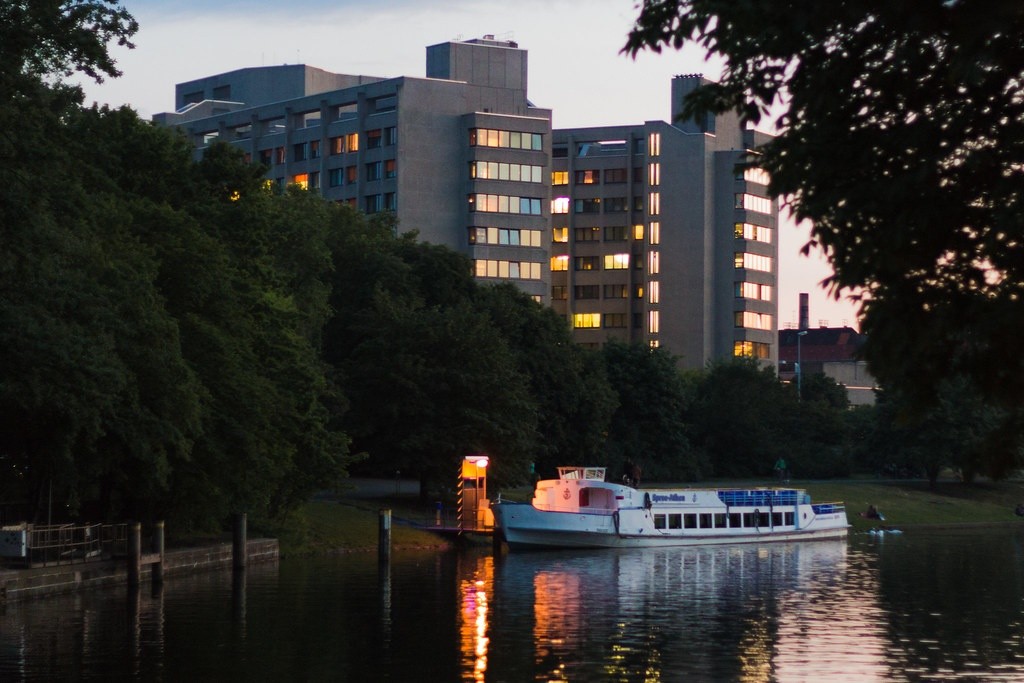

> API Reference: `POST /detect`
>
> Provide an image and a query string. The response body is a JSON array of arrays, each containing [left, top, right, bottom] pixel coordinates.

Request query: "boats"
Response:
[[489, 466, 853, 550]]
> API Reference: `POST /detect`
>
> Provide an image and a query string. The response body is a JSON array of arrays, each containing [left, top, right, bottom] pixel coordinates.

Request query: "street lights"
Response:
[[797, 331, 808, 404]]
